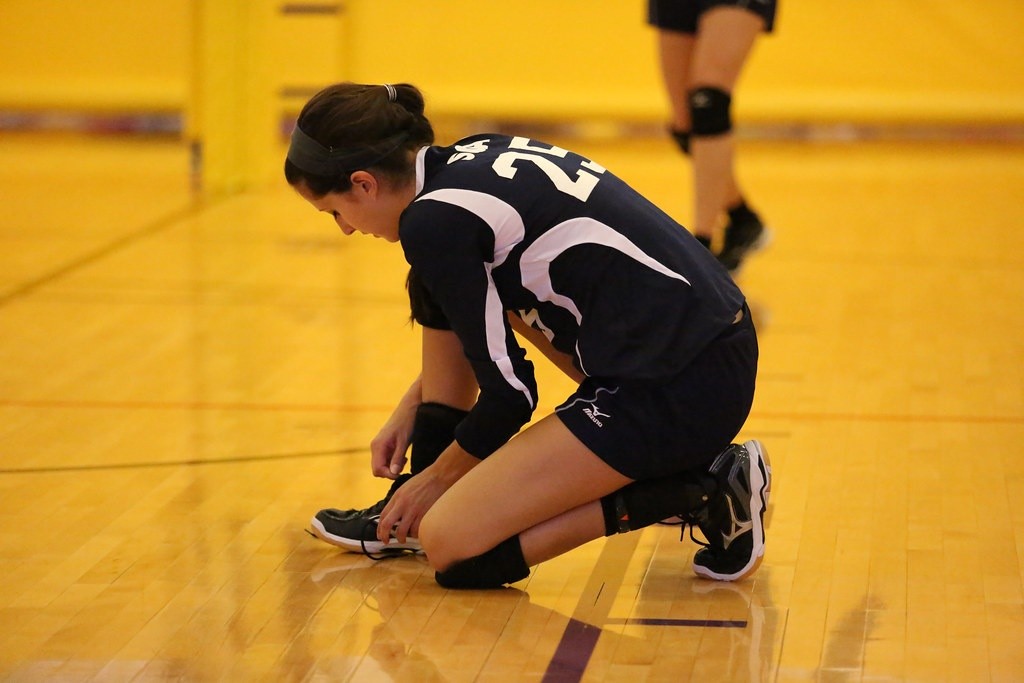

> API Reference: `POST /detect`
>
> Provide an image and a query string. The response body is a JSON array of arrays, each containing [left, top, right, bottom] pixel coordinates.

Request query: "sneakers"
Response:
[[685, 439, 771, 582], [310, 473, 435, 553]]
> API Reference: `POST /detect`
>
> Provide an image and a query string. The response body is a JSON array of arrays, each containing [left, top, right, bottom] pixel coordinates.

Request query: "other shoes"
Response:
[[692, 200, 770, 277]]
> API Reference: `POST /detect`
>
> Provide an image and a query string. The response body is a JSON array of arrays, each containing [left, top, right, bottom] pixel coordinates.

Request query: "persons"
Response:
[[647, 0, 778, 273], [285, 83, 772, 591]]
